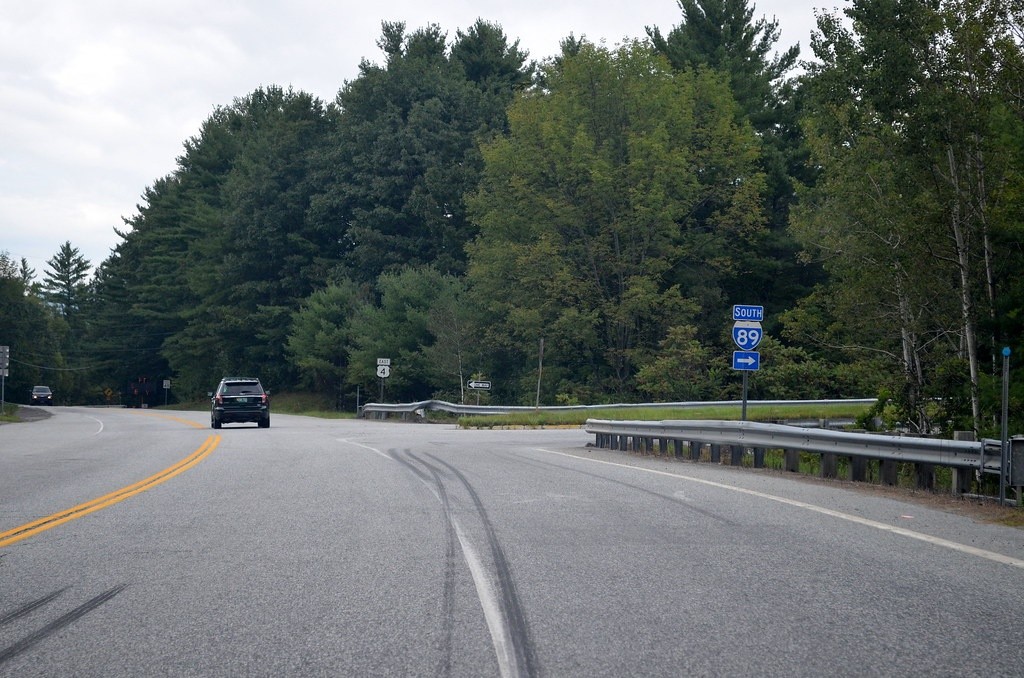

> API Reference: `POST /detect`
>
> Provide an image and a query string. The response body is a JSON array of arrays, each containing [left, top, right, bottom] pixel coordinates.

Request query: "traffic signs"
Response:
[[467, 380, 491, 390]]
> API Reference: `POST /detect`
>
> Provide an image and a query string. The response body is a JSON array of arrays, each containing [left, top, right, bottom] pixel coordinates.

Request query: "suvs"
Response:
[[208, 378, 270, 429], [29, 386, 54, 405]]
